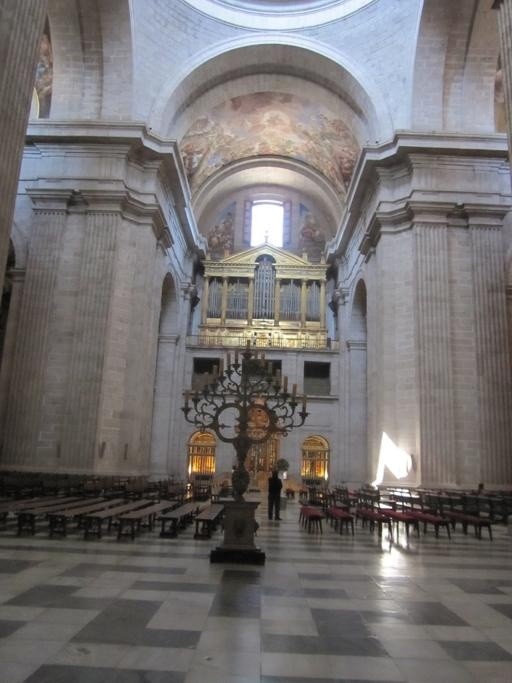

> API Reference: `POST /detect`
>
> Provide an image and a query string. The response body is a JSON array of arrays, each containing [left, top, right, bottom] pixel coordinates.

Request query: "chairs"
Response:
[[286, 481, 511, 542], [1, 469, 265, 543]]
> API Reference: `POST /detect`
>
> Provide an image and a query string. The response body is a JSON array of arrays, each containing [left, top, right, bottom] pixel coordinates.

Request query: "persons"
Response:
[[478, 482, 488, 497], [267, 468, 284, 520]]
